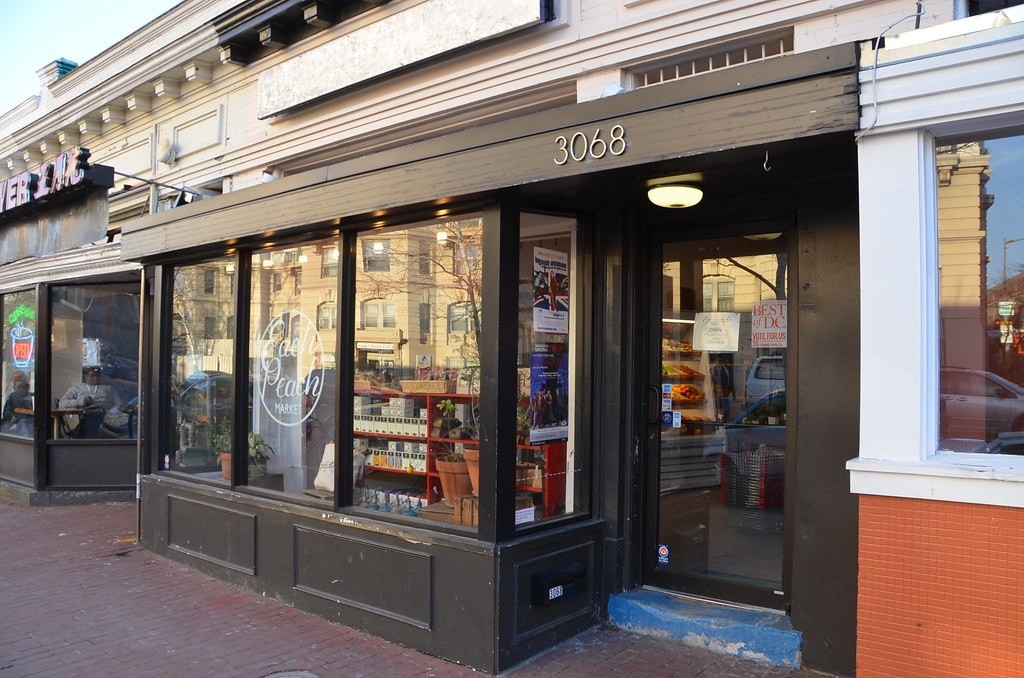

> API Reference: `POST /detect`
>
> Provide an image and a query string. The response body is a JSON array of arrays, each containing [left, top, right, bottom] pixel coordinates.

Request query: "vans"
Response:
[[744, 357, 785, 410]]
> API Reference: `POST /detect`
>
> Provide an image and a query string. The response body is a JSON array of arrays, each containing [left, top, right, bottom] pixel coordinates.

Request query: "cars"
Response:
[[940, 366, 1024, 440], [82, 353, 139, 382], [975, 430, 1024, 455], [703, 388, 786, 488], [304, 369, 336, 409], [179, 375, 313, 441], [99, 392, 142, 439]]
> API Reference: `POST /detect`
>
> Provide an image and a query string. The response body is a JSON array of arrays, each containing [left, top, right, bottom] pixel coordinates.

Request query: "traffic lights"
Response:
[[995, 319, 1013, 325]]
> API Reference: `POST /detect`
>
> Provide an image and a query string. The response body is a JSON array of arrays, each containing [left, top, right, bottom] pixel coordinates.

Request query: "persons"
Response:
[[709, 355, 737, 426], [0, 373, 35, 434], [534, 269, 569, 311], [531, 382, 567, 430], [57, 366, 124, 440]]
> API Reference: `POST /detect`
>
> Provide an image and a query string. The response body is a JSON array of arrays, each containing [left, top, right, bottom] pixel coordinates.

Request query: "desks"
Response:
[[15, 407, 84, 440]]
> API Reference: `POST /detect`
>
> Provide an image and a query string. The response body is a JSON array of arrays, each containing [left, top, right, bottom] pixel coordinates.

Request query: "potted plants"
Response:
[[460, 365, 479, 497], [429, 399, 472, 508]]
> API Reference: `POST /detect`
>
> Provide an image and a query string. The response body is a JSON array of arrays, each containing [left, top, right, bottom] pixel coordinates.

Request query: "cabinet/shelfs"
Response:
[[661, 310, 724, 494], [353, 390, 567, 519]]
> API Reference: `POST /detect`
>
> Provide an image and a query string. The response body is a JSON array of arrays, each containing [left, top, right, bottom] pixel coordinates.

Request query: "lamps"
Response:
[[648, 183, 703, 208]]
